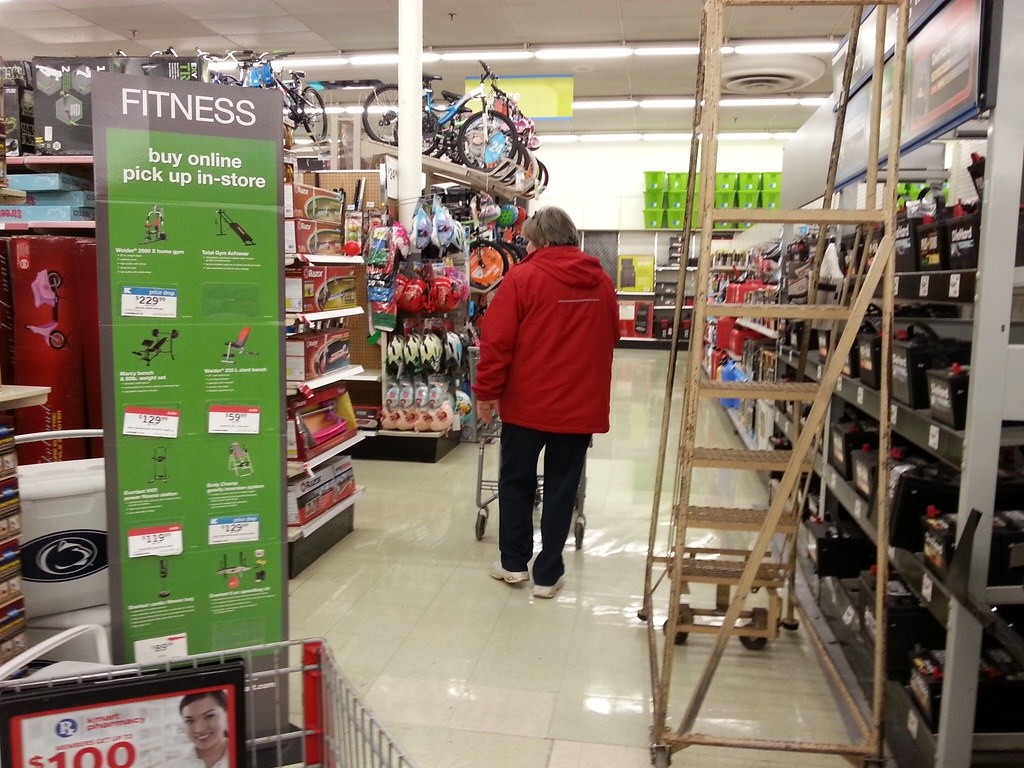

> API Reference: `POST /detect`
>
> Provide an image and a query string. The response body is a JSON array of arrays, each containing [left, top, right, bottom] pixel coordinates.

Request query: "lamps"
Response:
[[30, 33, 844, 108]]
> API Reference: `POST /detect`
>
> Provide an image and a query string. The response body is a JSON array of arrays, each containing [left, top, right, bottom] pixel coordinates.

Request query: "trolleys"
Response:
[[0, 637, 416, 768], [467, 345, 593, 551]]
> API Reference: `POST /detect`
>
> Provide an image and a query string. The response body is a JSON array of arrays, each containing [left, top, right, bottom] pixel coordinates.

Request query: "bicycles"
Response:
[[111, 45, 329, 144], [360, 59, 550, 201]]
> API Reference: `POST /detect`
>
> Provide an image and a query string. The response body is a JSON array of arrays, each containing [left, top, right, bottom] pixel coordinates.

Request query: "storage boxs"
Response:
[[3, 190, 95, 208], [0, 205, 95, 221], [616, 236, 698, 338], [283, 180, 356, 529], [643, 169, 782, 230], [6, 173, 93, 192]]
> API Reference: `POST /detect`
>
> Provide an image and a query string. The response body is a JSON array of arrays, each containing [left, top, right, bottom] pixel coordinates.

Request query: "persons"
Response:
[[179, 690, 228, 768], [472, 205, 621, 598]]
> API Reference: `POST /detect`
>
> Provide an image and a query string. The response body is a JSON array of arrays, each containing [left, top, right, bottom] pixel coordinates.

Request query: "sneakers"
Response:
[[490, 560, 531, 584], [533, 575, 565, 598]]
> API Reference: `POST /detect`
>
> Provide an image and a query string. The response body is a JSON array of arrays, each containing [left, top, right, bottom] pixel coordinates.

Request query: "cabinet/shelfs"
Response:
[[284, 252, 364, 580], [701, 265, 757, 387], [726, 315, 778, 451], [769, 268, 1024, 768], [618, 228, 747, 352], [0, 156, 96, 230]]
